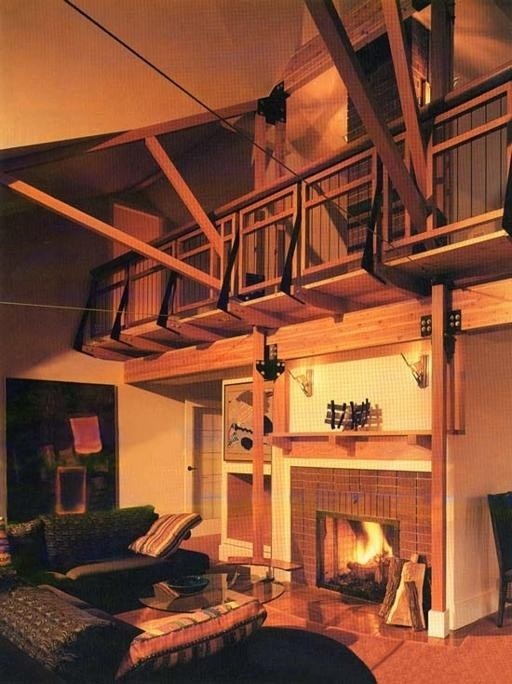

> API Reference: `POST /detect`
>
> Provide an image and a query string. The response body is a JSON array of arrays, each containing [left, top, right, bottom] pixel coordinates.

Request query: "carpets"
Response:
[[127, 626, 376, 684]]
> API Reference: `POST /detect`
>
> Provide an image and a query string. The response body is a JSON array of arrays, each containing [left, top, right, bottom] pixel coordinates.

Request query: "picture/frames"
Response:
[[223, 380, 272, 463]]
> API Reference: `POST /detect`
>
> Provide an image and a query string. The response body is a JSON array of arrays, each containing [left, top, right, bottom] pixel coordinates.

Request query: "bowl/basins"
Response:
[[166, 576, 210, 595]]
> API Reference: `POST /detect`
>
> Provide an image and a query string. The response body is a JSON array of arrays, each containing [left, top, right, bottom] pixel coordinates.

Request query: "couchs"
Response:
[[1, 505, 267, 684]]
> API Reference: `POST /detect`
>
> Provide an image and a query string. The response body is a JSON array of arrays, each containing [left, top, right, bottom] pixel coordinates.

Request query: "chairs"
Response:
[[486, 490, 512, 628]]
